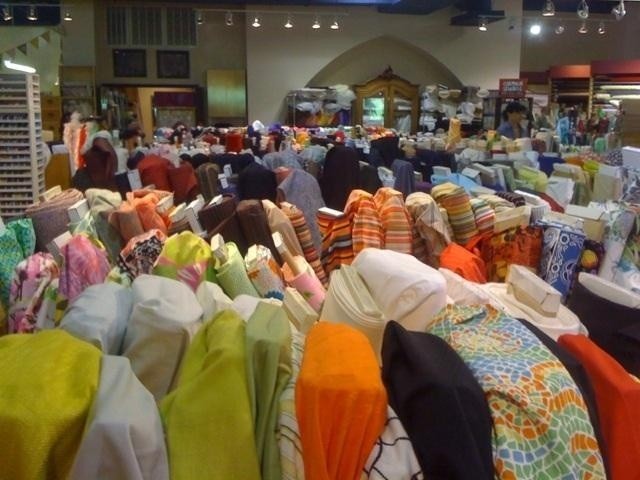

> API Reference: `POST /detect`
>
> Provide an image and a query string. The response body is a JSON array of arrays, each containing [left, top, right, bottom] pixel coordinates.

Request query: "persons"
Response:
[[497, 102, 529, 140]]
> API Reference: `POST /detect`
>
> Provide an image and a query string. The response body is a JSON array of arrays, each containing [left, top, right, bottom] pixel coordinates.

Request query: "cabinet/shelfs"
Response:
[[58, 64, 98, 125], [39, 95, 62, 143], [200, 69, 247, 119]]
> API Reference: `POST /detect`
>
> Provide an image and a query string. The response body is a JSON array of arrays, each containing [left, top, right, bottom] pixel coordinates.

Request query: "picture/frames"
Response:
[[111, 47, 147, 78], [155, 49, 190, 78]]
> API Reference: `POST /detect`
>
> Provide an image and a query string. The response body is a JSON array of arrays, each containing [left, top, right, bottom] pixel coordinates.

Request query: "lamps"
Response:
[[193, 7, 344, 31], [540, 0, 628, 21], [0, 2, 73, 24], [477, 13, 608, 35]]
